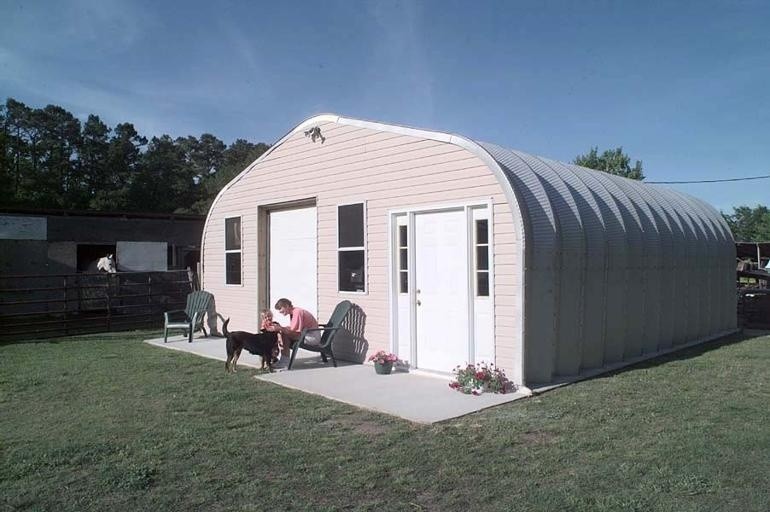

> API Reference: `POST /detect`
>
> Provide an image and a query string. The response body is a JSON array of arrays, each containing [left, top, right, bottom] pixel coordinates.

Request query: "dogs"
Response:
[[221, 318, 280, 375]]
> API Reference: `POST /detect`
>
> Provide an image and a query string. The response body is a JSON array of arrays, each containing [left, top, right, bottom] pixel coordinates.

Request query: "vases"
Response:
[[468, 379, 488, 396], [374, 362, 392, 375]]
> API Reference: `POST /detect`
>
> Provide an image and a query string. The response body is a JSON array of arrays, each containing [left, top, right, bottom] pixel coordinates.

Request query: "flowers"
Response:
[[368, 350, 397, 364], [449, 363, 508, 397]]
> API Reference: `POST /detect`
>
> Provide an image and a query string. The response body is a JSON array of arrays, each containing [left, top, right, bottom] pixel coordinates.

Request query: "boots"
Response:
[[267, 350, 291, 371]]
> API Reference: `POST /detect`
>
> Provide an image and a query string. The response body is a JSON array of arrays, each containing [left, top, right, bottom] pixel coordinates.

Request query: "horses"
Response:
[[78, 253, 117, 274]]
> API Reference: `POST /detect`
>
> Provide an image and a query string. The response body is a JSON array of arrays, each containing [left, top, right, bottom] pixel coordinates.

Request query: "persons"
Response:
[[260, 309, 290, 363], [97, 250, 117, 273], [264, 298, 321, 370]]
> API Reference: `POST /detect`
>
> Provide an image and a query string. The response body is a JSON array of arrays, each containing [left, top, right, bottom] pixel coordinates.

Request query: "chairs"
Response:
[[288, 300, 352, 369], [163, 290, 214, 343]]
